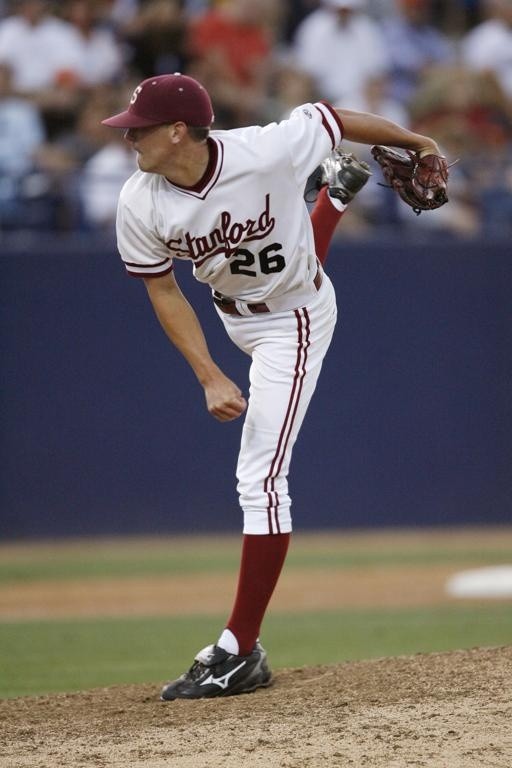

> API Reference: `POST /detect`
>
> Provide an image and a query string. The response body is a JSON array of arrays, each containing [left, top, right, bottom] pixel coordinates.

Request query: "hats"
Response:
[[101, 72, 213, 129]]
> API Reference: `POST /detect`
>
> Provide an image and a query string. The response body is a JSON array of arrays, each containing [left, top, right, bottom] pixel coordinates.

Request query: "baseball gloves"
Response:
[[370, 145, 449, 211]]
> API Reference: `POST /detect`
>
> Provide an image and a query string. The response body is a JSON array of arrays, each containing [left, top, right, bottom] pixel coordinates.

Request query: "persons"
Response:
[[1, 1, 512, 234], [103, 68, 454, 702]]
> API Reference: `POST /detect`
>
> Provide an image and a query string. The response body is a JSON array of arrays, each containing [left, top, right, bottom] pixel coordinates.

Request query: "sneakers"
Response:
[[304, 144, 373, 205], [160, 628, 275, 702]]
